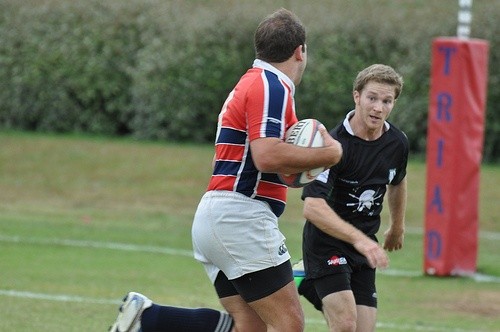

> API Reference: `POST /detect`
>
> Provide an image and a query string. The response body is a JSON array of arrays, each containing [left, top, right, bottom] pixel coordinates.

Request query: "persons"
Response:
[[107, 10, 342, 332], [300, 64, 409, 332]]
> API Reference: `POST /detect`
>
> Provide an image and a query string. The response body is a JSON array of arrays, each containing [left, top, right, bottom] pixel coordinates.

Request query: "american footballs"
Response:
[[277, 118, 328, 189]]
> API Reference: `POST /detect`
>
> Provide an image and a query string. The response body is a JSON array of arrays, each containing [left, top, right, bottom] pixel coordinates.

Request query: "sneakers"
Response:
[[108, 292, 152, 332]]
